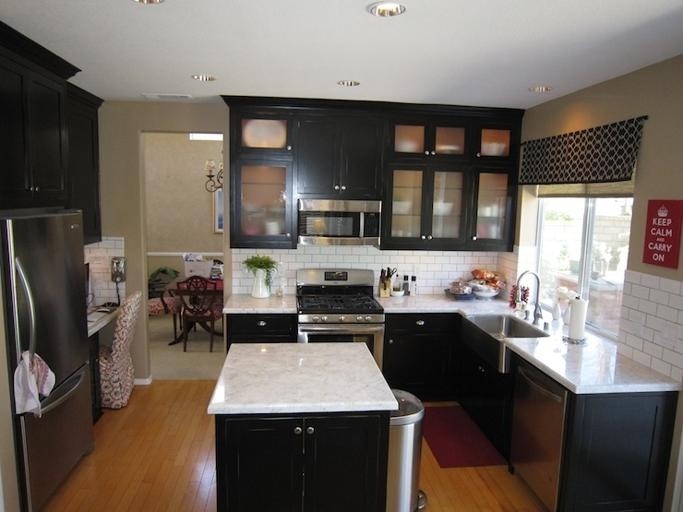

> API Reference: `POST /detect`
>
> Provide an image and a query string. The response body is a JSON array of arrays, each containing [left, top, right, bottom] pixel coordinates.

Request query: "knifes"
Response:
[[380, 267, 392, 289]]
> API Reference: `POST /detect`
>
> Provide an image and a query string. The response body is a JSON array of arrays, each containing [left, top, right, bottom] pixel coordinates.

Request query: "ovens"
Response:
[[298, 321, 386, 373]]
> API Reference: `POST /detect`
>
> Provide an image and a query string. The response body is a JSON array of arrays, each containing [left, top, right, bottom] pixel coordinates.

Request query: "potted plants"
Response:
[[242, 255, 279, 297]]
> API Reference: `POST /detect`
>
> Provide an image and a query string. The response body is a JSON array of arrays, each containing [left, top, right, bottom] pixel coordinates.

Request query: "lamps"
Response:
[[202, 141, 224, 193], [102, 256, 128, 308]]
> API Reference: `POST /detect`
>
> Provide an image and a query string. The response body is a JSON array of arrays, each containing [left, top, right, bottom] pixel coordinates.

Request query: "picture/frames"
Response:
[[212, 183, 223, 235]]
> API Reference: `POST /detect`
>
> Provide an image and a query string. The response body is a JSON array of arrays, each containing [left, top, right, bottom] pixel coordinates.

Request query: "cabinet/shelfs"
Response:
[[382, 312, 458, 402], [458, 314, 515, 464], [67, 80, 105, 246], [471, 105, 525, 252], [381, 100, 472, 252], [220, 93, 298, 249], [0, 20, 82, 211], [298, 98, 381, 201], [223, 313, 298, 353], [207, 410, 399, 512]]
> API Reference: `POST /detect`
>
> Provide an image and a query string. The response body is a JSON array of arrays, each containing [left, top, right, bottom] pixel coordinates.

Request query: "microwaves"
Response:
[[297, 200, 382, 247]]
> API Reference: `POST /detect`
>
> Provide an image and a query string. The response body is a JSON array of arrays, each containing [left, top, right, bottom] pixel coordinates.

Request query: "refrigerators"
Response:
[[1, 206, 97, 512]]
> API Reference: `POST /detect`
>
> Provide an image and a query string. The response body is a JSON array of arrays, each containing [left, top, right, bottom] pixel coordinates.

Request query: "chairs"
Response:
[[175, 277, 216, 352], [98, 290, 144, 409], [147, 282, 182, 339]]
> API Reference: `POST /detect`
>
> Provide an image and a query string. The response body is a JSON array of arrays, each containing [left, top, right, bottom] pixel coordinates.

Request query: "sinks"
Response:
[[465, 313, 551, 342]]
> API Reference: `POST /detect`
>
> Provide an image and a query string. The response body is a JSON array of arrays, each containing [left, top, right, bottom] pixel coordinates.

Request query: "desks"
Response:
[[87, 300, 124, 426], [165, 275, 223, 338]]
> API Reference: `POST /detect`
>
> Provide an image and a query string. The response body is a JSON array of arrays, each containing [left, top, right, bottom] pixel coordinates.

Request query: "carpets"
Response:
[[420, 407, 508, 469]]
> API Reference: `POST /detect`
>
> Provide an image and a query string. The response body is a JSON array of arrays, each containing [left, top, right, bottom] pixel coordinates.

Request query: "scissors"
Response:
[[389, 268, 397, 277]]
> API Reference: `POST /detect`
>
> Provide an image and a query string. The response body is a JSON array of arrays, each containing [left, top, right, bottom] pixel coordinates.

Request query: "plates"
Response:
[[435, 144, 461, 153]]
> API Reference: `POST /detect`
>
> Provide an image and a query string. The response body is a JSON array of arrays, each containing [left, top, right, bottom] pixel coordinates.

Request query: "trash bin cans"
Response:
[[385, 388, 427, 512]]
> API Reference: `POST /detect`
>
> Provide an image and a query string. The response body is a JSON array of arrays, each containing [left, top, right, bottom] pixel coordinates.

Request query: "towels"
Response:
[[13, 350, 57, 419]]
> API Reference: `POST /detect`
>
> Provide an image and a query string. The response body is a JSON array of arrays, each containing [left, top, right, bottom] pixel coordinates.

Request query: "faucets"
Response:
[[513, 270, 543, 325]]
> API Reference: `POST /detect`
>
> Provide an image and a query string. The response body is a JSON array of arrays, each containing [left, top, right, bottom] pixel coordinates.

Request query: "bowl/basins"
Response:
[[392, 200, 411, 215], [432, 202, 454, 216], [482, 141, 505, 155]]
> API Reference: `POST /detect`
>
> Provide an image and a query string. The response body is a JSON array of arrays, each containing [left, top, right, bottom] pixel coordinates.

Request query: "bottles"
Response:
[[393, 274, 417, 297]]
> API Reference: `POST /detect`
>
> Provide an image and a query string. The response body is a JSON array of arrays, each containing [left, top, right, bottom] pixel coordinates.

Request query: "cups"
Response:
[[481, 204, 499, 217]]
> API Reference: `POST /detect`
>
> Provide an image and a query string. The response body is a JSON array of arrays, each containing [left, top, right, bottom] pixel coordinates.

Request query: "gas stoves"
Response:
[[297, 289, 384, 324]]
